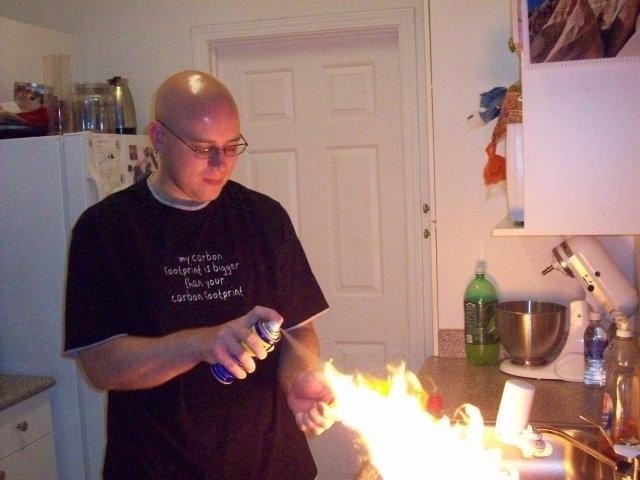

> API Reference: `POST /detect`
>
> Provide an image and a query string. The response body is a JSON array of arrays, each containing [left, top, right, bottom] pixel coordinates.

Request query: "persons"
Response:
[[59, 69, 339, 479]]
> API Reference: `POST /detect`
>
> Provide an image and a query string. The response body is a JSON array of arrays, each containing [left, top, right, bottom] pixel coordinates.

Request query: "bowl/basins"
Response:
[[13, 80, 50, 113]]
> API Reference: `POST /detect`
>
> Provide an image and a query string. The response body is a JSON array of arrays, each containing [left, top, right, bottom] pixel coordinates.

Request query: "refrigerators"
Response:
[[1, 132, 159, 480]]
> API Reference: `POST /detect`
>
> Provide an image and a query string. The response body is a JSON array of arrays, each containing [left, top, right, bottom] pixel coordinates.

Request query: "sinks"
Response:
[[357, 421, 618, 480]]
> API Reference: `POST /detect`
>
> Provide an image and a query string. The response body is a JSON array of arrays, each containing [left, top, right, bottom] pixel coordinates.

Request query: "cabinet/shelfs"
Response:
[[492, 1, 640, 242], [0, 390, 57, 479]]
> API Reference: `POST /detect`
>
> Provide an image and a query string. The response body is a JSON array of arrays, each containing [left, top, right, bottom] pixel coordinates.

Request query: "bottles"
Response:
[[463, 268, 498, 363], [494, 237, 638, 382], [583, 312, 608, 387], [71, 82, 101, 132]]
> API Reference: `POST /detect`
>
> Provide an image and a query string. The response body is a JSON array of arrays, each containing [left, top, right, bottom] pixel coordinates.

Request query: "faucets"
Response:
[[532, 426, 634, 474]]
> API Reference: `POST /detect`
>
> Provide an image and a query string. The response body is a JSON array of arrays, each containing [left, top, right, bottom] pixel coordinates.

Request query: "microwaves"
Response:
[[505, 122, 524, 227]]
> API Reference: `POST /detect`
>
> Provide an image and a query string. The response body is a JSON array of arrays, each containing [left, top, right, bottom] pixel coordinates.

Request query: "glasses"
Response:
[[154, 116, 248, 160]]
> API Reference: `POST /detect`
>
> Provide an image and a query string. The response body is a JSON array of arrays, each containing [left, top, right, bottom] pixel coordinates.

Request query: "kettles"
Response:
[[101, 76, 137, 135]]
